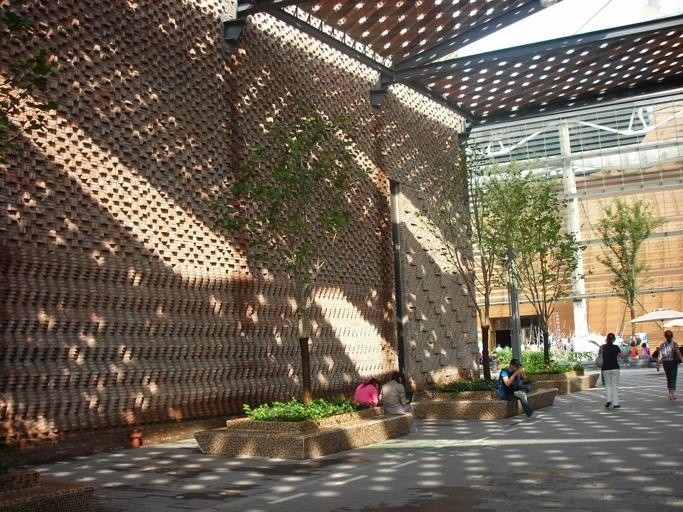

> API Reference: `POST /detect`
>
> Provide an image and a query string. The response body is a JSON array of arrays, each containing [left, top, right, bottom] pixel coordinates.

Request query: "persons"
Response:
[[496, 359, 533, 417], [487, 338, 574, 355], [621, 334, 682, 363], [597, 332, 623, 409], [379, 372, 418, 432], [352, 377, 378, 407], [656, 330, 682, 400]]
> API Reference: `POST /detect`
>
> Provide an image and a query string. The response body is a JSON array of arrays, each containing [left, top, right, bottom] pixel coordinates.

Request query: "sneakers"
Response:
[[605, 402, 611, 407], [669, 394, 677, 400], [528, 414, 541, 420], [513, 391, 526, 399], [614, 406, 620, 407]]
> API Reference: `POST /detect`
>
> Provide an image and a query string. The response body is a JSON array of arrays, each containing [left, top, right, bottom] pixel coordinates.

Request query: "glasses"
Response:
[[513, 364, 519, 368]]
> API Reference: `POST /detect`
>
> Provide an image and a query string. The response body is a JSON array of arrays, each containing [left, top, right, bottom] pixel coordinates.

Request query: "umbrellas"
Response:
[[627, 306, 683, 323], [662, 319, 683, 327]]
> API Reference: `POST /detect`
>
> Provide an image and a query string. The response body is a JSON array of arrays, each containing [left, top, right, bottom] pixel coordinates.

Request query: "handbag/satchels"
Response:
[[648, 351, 649, 355], [673, 351, 681, 363], [503, 384, 515, 394], [596, 354, 603, 368]]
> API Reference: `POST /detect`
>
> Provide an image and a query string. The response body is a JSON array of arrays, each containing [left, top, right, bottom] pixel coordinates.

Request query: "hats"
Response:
[[511, 359, 520, 365]]
